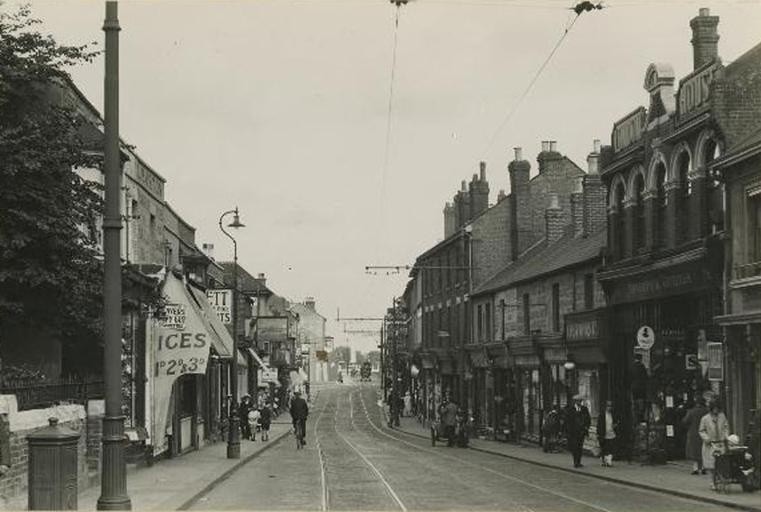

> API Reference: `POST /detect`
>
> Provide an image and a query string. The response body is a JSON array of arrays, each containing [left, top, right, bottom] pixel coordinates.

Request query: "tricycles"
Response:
[[431, 411, 469, 447]]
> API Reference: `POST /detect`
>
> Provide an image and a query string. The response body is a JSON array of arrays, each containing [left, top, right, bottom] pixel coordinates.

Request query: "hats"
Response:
[[726, 435, 739, 444], [572, 394, 582, 400]]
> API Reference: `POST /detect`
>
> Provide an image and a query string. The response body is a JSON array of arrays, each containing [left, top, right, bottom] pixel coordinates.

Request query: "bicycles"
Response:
[[294, 417, 306, 449]]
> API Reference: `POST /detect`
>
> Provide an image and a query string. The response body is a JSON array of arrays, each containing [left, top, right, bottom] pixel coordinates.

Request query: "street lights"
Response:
[[221, 208, 245, 458]]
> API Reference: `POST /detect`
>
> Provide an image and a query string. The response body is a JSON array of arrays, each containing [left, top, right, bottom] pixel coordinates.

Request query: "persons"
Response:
[[564, 395, 591, 468], [594, 401, 623, 466], [385, 391, 402, 427], [724, 433, 752, 464], [440, 395, 457, 447], [289, 391, 309, 444], [679, 402, 707, 476], [698, 400, 731, 469], [437, 397, 449, 416], [238, 386, 272, 442], [541, 404, 561, 452]]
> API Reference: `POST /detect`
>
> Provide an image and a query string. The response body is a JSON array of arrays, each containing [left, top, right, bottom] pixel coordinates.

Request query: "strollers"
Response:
[[709, 435, 760, 493]]
